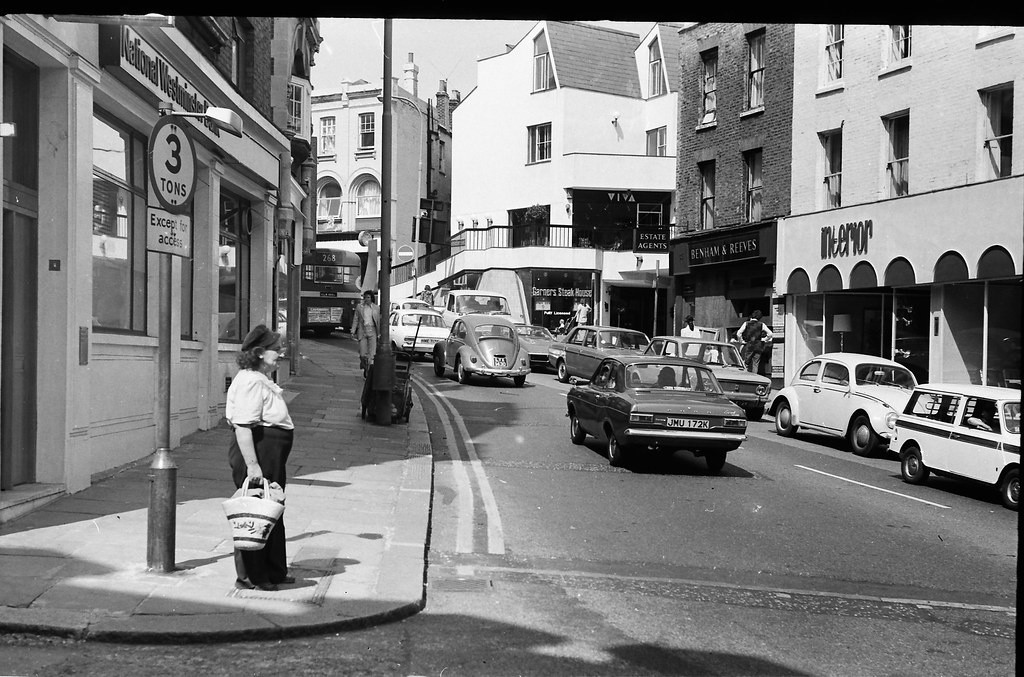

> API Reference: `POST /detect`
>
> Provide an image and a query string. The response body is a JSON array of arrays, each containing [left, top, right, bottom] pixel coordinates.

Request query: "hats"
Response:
[[241, 324, 281, 350]]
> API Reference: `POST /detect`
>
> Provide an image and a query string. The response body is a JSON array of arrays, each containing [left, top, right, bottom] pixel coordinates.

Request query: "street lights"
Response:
[[378, 93, 420, 300], [145, 100, 246, 568]]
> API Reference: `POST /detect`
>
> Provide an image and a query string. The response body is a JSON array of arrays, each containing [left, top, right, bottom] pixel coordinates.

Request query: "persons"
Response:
[[736, 310, 773, 374], [318, 268, 332, 281], [465, 295, 480, 305], [350, 289, 381, 381], [572, 297, 592, 340], [488, 301, 503, 312], [420, 285, 434, 306], [225, 324, 296, 591], [680, 315, 702, 356], [654, 367, 677, 388], [966, 403, 1000, 434], [551, 319, 565, 332]]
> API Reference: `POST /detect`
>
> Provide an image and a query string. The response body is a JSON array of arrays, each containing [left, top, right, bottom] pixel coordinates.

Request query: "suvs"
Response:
[[888, 386, 1020, 512]]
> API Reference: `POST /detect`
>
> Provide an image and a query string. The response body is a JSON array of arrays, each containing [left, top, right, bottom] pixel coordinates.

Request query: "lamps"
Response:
[[566, 204, 570, 211], [612, 114, 621, 127], [635, 255, 643, 266]]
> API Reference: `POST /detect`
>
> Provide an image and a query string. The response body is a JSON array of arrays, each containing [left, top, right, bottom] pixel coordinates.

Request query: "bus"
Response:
[[300, 247, 363, 338]]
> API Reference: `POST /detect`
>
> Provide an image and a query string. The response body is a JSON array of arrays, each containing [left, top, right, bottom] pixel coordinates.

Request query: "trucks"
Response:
[[447, 292, 511, 321]]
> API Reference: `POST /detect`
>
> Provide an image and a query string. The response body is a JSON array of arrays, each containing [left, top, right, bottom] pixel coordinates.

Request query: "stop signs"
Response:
[[398, 245, 415, 262]]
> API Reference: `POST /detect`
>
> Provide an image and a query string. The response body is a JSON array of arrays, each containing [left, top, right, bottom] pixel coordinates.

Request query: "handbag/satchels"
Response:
[[226, 476, 285, 550]]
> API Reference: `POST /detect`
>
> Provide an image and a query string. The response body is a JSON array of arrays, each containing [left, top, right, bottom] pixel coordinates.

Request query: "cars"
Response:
[[549, 321, 656, 383], [569, 356, 747, 471], [645, 337, 771, 423], [391, 299, 429, 314], [432, 314, 534, 386], [391, 308, 450, 356], [769, 353, 918, 457], [513, 324, 555, 372]]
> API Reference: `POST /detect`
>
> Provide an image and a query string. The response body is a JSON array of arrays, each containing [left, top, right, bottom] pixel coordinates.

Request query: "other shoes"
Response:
[[236, 575, 295, 591]]
